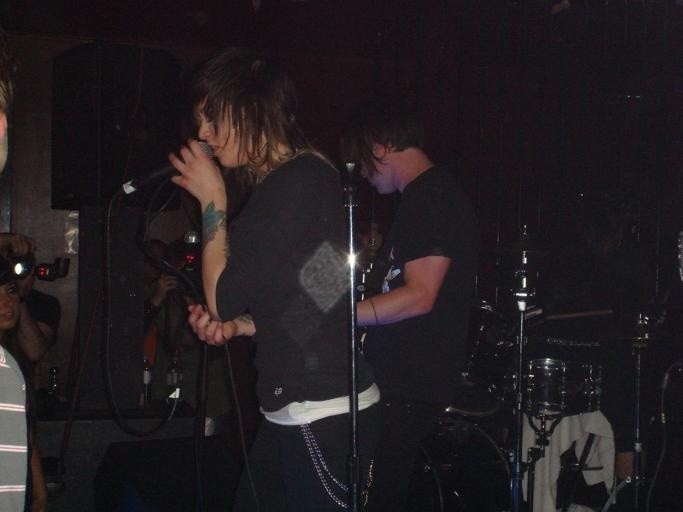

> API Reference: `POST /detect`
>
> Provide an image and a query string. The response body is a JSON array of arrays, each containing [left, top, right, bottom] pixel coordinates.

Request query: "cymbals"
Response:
[[607, 337, 674, 351]]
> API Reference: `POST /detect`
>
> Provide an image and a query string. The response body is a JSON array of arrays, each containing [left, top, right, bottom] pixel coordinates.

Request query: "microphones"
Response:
[[122, 141, 213, 195]]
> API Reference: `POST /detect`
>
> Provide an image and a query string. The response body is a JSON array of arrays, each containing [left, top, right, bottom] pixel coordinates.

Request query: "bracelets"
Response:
[[366, 295, 380, 330]]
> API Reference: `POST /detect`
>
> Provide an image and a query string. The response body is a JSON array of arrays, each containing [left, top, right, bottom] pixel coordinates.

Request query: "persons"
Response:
[[0, 233, 64, 386], [350, 211, 397, 299], [0, 32, 34, 512], [339, 94, 484, 508], [0, 255, 50, 511], [177, 39, 388, 510], [127, 258, 238, 418]]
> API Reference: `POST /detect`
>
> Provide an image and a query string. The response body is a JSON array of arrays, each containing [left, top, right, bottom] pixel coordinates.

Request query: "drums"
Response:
[[516, 360, 600, 417], [447, 304, 525, 417], [404, 422, 515, 511]]
